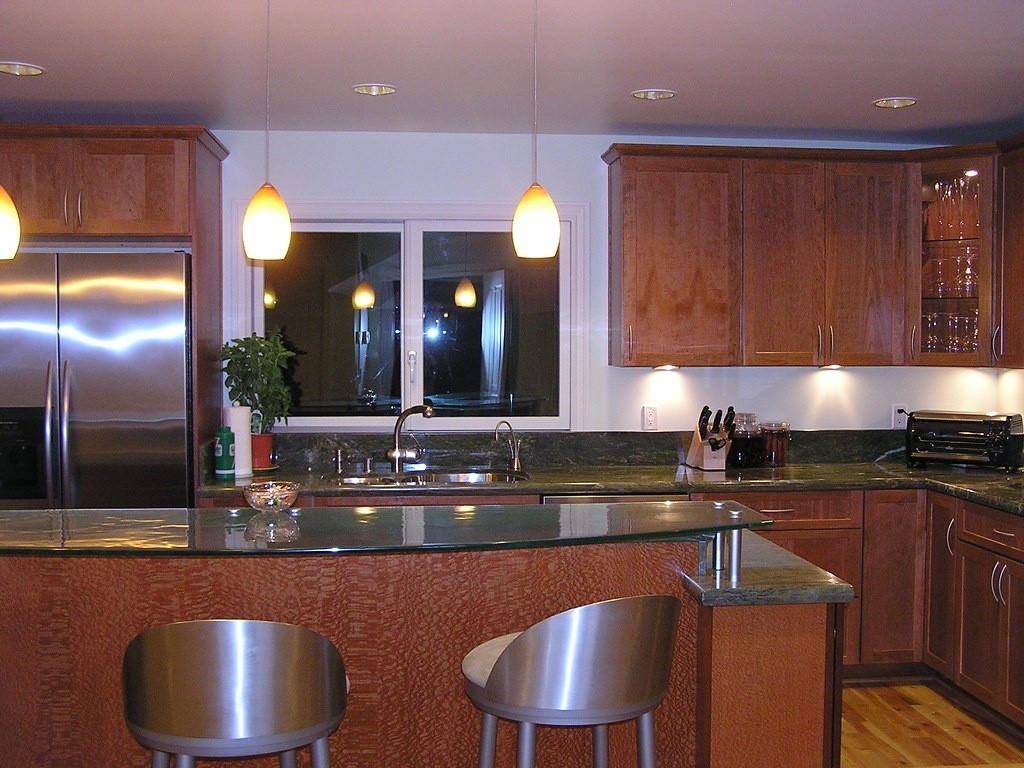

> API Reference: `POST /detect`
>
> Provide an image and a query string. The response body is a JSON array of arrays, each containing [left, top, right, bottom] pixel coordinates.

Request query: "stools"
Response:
[[123, 619, 352, 768], [461, 593, 681, 768]]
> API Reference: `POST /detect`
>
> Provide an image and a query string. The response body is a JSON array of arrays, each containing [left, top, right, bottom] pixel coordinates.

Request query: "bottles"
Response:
[[215, 427, 235, 480], [762, 422, 790, 466], [731, 413, 761, 465]]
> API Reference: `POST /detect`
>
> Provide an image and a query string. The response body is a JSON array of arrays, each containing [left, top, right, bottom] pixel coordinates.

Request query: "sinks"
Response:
[[334, 477, 398, 488], [401, 471, 529, 487]]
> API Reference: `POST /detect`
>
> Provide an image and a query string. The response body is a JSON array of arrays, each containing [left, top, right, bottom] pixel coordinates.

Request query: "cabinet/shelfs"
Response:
[[702, 491, 1024, 734], [601, 141, 1024, 369], [0, 121, 228, 244]]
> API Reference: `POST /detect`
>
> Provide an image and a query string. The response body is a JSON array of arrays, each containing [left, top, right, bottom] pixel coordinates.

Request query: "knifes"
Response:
[[697, 406, 736, 438]]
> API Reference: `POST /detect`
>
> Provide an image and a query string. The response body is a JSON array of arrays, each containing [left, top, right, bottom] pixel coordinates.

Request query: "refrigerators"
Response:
[[0, 248, 191, 510]]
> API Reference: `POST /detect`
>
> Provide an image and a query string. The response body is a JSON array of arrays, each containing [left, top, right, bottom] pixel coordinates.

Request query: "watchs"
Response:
[[449, 373, 451, 375]]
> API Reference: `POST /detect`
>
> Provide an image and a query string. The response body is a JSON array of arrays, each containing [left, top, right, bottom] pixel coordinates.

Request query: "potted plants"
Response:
[[217, 327, 296, 468]]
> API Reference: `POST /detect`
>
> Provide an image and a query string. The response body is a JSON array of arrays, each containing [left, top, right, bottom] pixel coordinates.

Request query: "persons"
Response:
[[424, 281, 454, 398]]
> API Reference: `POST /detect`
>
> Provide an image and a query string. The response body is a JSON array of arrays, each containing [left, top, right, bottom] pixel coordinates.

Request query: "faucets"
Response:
[[494, 420, 523, 470], [385, 404, 435, 472]]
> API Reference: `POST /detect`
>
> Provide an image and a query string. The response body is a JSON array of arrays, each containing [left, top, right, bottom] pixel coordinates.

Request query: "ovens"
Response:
[[907, 410, 1024, 472]]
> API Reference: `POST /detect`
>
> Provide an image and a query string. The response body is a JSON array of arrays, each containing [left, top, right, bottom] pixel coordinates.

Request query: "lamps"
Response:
[[242, 0, 291, 261], [455, 234, 476, 308], [511, 0, 561, 261], [264, 283, 277, 308], [353, 268, 375, 308]]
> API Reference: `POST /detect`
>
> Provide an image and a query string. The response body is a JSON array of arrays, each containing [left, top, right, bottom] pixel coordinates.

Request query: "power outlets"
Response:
[[891, 404, 908, 429], [643, 406, 658, 430]]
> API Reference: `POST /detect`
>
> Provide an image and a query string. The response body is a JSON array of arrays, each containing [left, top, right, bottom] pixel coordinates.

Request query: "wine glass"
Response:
[[923, 176, 978, 353]]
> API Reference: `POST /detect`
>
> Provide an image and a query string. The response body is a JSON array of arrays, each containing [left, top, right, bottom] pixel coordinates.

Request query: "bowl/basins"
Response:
[[242, 484, 298, 512], [245, 513, 301, 541]]
